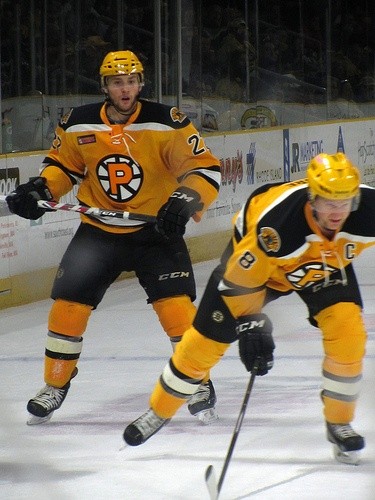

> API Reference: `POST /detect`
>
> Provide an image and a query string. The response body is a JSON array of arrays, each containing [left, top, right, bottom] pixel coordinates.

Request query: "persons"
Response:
[[6, 50, 221, 426], [118, 153, 375, 466]]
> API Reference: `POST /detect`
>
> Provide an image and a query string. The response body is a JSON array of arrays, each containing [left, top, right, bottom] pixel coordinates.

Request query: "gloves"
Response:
[[156, 185, 204, 241], [5, 177, 57, 220], [235, 313, 275, 376]]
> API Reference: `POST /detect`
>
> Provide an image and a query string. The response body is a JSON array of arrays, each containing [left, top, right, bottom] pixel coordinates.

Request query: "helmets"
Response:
[[306, 152, 360, 199], [100, 50, 144, 76]]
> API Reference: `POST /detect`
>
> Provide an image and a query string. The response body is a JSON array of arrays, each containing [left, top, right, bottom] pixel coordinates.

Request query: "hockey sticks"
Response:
[[204, 362, 259, 499], [0, 193, 159, 224]]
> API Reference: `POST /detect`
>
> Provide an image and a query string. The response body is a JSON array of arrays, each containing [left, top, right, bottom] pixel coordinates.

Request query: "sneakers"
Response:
[[119, 407, 171, 452], [188, 379, 217, 425], [326, 421, 364, 465], [26, 366, 78, 425]]
